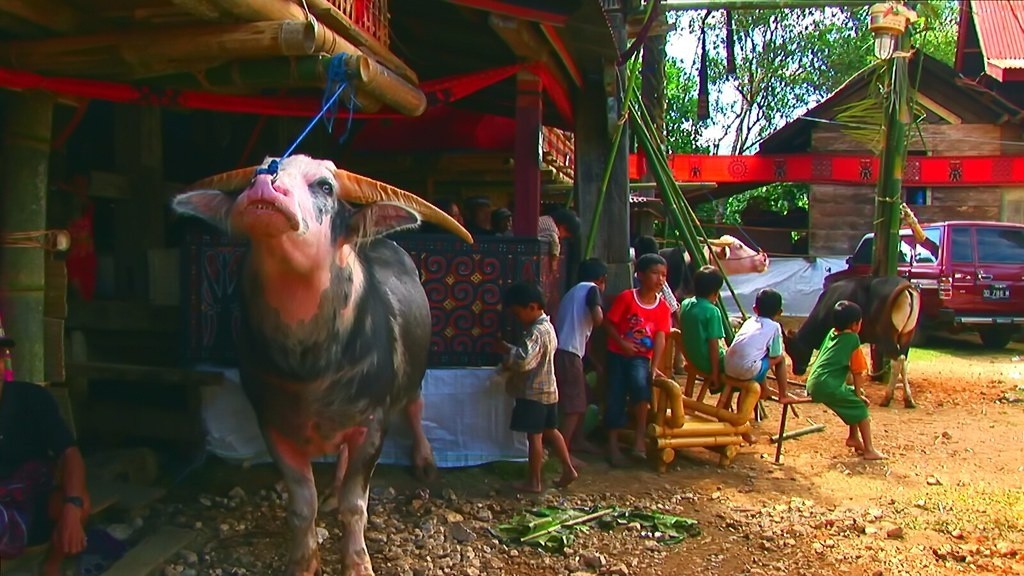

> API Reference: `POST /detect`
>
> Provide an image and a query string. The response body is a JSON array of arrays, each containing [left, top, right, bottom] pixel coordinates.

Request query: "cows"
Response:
[[172, 153, 474, 576], [780, 275, 921, 408], [627, 230, 769, 375]]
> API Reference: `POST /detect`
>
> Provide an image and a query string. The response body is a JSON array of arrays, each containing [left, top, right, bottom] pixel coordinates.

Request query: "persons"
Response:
[[0, 379, 88, 576], [554, 258, 609, 468], [680, 265, 741, 396], [467, 205, 525, 258], [724, 288, 800, 404], [537, 209, 581, 242], [806, 300, 890, 460], [603, 253, 672, 468], [633, 236, 681, 327], [491, 281, 578, 492], [432, 199, 464, 225]]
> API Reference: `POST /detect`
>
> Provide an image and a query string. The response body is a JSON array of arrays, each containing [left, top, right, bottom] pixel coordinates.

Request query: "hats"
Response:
[[492, 209, 512, 226], [0, 324, 15, 346]]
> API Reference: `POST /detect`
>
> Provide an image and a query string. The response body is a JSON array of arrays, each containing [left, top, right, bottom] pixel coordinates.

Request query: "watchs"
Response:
[[63, 496, 84, 508]]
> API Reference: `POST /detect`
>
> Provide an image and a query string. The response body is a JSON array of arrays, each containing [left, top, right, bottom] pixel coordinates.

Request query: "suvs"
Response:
[[822, 220, 1024, 348]]
